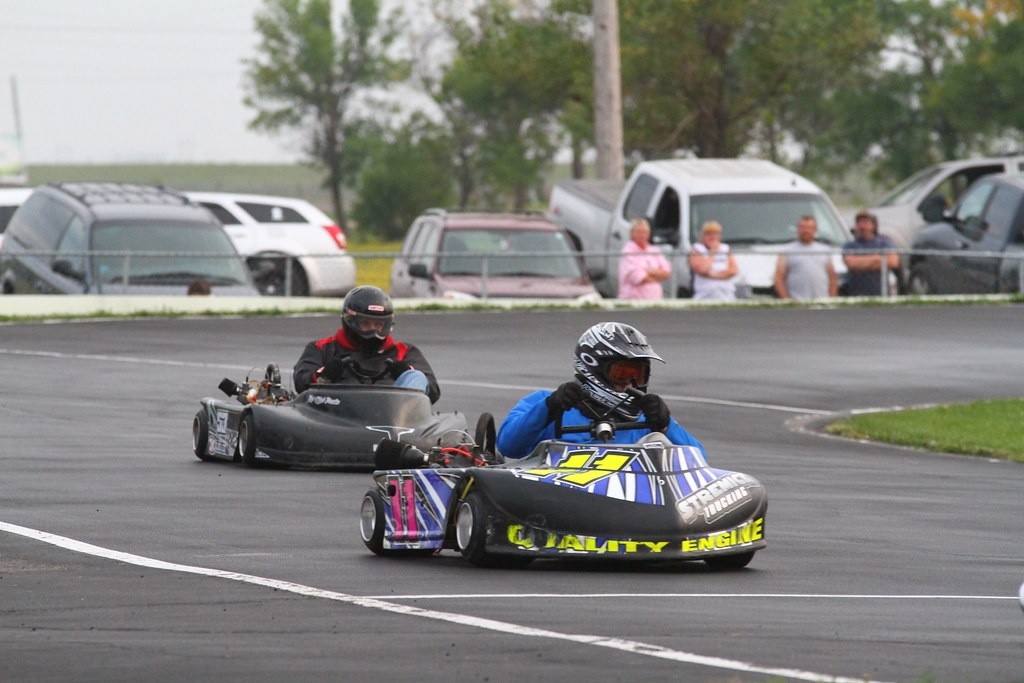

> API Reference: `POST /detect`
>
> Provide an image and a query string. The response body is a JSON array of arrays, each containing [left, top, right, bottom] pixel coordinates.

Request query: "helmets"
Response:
[[573, 322, 667, 422], [341, 285, 396, 355]]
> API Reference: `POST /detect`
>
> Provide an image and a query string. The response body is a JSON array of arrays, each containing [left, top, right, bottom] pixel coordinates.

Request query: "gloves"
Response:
[[387, 360, 412, 382], [636, 394, 672, 432], [319, 354, 347, 382], [547, 382, 585, 414]]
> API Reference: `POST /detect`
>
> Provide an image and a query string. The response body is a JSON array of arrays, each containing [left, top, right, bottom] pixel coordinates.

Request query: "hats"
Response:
[[628, 218, 651, 232], [701, 220, 722, 232]]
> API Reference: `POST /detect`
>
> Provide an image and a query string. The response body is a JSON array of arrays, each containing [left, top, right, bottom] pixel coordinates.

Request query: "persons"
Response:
[[187, 279, 210, 296], [293, 285, 439, 406], [841, 210, 900, 299], [616, 219, 671, 299], [497, 320, 710, 467], [689, 220, 739, 298], [775, 215, 837, 298]]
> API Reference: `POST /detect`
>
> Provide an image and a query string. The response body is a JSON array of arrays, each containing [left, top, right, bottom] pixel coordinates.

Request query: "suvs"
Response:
[[2, 180, 264, 297], [390, 207, 604, 302], [175, 190, 357, 295]]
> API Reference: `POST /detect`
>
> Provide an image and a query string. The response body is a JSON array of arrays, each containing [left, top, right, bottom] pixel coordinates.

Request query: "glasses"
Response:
[[596, 358, 650, 386]]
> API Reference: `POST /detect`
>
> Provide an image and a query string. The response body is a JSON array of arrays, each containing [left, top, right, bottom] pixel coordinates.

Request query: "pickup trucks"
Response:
[[545, 147, 858, 299]]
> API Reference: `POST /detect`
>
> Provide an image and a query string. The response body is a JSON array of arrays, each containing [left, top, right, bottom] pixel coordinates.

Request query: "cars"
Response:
[[843, 156, 1024, 284], [905, 171, 1024, 295]]
